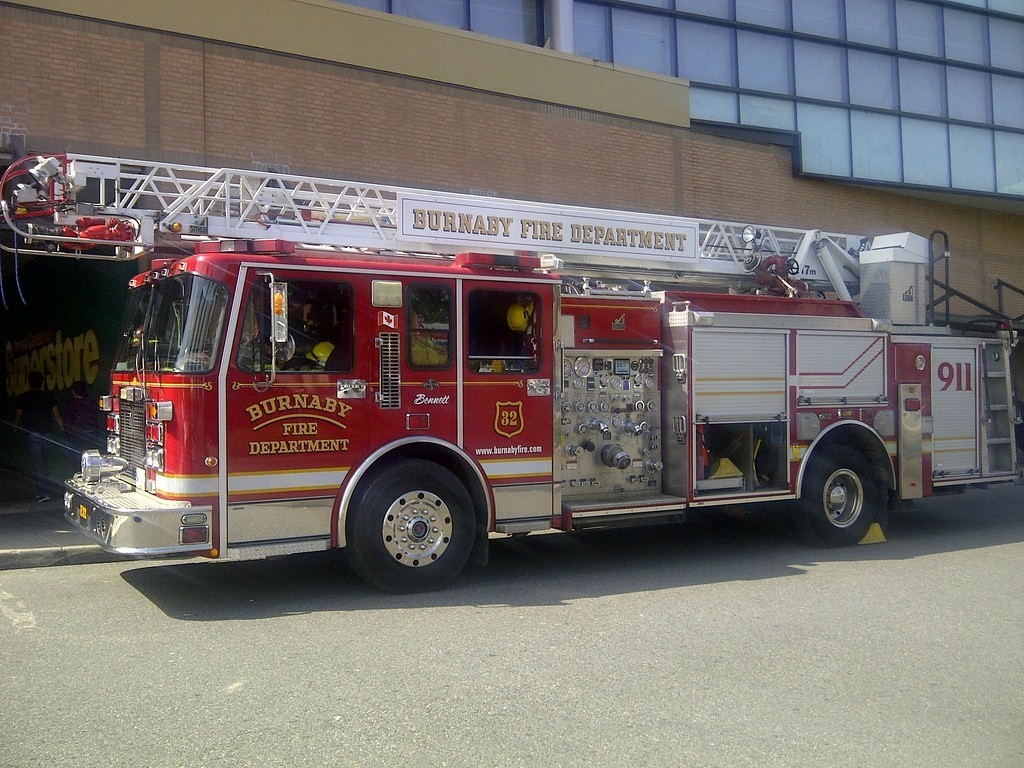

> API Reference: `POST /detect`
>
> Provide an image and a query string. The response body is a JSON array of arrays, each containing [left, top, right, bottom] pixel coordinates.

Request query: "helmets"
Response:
[[507, 301, 533, 331], [306, 342, 333, 367]]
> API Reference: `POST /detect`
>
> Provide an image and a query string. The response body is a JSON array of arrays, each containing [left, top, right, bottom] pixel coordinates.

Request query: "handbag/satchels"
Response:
[[64, 438, 83, 464]]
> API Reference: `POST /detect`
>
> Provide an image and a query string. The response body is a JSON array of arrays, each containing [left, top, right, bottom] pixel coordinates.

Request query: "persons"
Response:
[[61, 381, 100, 476], [12, 370, 65, 504]]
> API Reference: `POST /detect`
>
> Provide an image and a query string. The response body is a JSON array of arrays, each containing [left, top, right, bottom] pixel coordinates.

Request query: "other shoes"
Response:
[[37, 496, 50, 502], [32, 495, 39, 501]]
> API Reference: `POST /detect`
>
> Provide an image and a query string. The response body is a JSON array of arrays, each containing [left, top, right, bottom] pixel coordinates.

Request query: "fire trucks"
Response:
[[1, 153, 1024, 596]]
